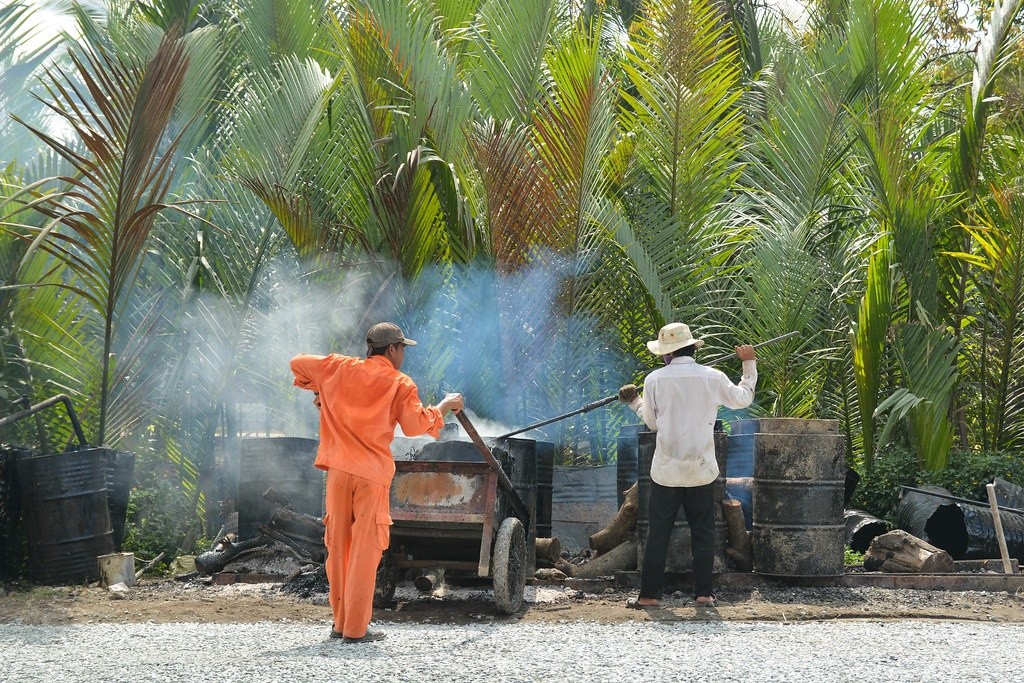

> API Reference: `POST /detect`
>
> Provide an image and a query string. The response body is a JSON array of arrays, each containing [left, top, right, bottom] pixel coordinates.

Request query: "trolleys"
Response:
[[316, 401, 531, 616]]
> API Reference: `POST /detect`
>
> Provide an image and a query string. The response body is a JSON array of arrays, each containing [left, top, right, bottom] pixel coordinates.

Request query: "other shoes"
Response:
[[329, 624, 343, 638], [343, 631, 387, 643]]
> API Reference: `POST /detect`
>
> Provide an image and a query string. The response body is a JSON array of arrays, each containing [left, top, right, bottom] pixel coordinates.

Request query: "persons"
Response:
[[291, 321, 463, 644], [620, 322, 758, 609]]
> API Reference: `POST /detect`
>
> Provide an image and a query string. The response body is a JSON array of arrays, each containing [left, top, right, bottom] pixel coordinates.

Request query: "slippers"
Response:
[[696, 592, 718, 607], [625, 595, 659, 610]]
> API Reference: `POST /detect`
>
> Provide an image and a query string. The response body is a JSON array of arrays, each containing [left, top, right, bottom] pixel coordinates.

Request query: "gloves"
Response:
[[618, 383, 637, 404]]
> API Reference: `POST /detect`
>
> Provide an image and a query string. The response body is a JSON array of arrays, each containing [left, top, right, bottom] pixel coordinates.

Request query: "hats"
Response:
[[366, 322, 417, 358], [647, 322, 706, 356]]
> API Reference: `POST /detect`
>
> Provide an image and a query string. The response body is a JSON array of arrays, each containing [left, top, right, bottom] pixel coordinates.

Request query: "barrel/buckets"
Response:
[[387, 438, 617, 542], [618, 416, 848, 576], [5, 444, 136, 587], [240, 438, 323, 535]]
